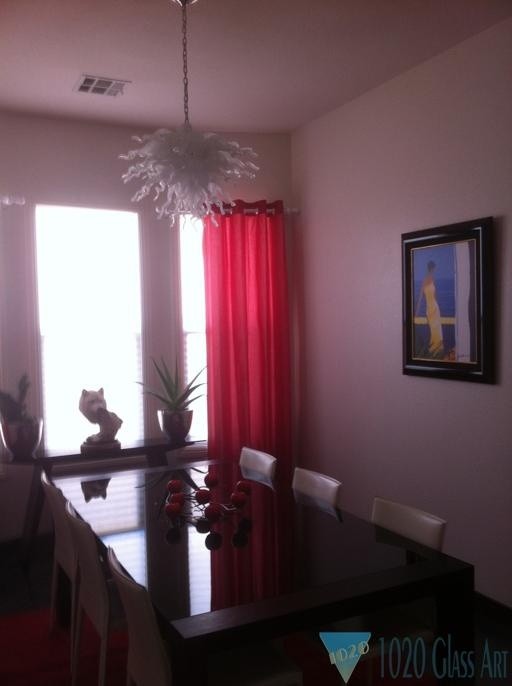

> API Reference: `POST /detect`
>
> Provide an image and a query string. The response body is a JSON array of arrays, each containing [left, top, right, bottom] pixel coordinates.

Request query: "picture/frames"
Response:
[[401, 216, 496, 385]]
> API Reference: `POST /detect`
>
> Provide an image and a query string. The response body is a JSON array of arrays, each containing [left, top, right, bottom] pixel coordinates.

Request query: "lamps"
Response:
[[117, 0, 262, 229]]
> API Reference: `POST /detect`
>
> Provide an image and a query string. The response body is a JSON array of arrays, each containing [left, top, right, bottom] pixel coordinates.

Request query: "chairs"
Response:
[[65, 511, 127, 686], [372, 496, 447, 552], [239, 447, 277, 476], [290, 467, 342, 504], [40, 468, 72, 637], [107, 544, 199, 685]]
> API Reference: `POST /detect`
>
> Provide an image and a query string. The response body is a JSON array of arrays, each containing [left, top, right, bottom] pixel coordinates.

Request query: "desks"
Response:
[[50, 456, 476, 684]]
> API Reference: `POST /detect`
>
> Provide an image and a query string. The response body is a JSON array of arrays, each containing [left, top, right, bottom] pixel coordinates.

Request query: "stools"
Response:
[[284, 614, 436, 684]]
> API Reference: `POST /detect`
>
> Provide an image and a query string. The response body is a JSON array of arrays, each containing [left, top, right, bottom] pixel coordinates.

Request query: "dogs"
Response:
[[79, 388, 106, 422]]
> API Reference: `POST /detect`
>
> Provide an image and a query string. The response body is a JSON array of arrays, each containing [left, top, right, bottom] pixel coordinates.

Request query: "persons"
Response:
[[414, 261, 444, 358]]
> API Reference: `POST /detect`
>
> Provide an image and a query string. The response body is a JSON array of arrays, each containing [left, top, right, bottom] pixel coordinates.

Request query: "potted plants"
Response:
[[1, 374, 45, 462], [133, 354, 208, 442]]
